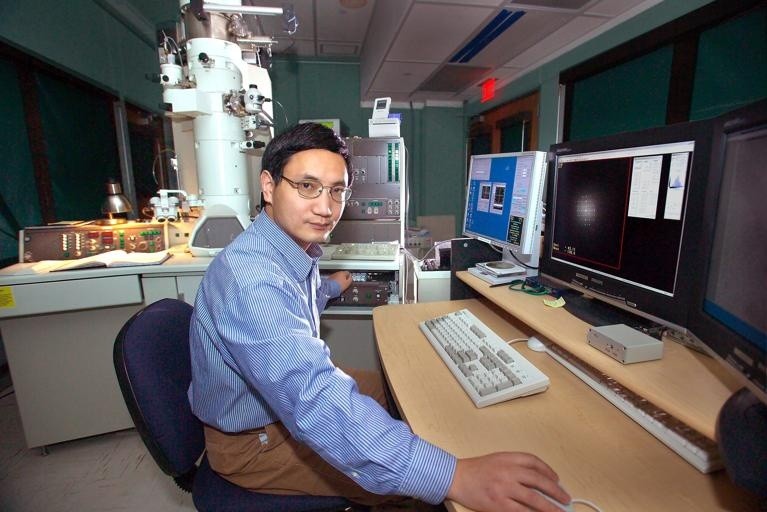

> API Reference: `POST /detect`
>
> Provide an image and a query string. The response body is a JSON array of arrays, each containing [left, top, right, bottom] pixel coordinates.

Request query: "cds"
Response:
[[486, 262, 515, 269]]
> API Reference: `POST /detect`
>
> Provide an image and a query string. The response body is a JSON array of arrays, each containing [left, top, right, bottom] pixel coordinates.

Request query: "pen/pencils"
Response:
[[489, 279, 523, 288]]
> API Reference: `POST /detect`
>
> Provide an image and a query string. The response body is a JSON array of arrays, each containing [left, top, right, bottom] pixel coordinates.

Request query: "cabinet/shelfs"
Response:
[[0, 268, 177, 456], [172, 263, 402, 373]]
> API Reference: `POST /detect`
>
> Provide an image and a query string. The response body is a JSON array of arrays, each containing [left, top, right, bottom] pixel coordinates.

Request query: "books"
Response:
[[50, 250, 174, 272]]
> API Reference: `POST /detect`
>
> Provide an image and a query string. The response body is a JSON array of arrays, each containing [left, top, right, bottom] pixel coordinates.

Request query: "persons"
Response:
[[186, 122, 572, 512]]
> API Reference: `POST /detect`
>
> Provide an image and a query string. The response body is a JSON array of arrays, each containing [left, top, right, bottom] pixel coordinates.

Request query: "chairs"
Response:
[[112, 298, 376, 512]]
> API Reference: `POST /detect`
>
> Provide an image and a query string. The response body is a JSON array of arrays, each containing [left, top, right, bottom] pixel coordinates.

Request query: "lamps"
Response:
[[95, 180, 133, 226]]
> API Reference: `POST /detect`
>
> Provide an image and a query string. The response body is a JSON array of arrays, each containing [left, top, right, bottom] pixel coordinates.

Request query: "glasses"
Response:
[[273, 173, 354, 203]]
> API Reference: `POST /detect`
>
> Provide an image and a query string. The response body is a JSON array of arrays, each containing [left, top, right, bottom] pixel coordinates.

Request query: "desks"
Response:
[[372, 269, 765, 511]]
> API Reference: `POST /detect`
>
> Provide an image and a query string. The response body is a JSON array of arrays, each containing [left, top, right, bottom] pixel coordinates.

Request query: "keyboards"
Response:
[[547, 344, 728, 474], [419, 308, 549, 408]]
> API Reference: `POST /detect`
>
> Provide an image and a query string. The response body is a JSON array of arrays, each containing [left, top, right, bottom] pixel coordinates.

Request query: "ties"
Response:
[[309, 263, 323, 341]]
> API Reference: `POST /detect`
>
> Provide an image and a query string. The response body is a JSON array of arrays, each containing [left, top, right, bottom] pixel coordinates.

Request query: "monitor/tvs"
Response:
[[539, 120, 712, 329], [463, 151, 547, 287], [690, 98, 766, 393]]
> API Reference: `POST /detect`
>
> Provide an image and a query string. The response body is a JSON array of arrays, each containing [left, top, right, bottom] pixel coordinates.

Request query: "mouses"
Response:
[[528, 482, 575, 512], [528, 332, 552, 354]]
